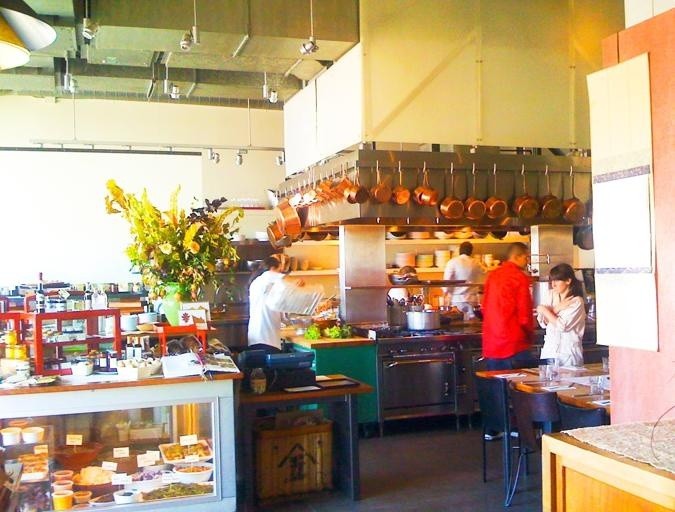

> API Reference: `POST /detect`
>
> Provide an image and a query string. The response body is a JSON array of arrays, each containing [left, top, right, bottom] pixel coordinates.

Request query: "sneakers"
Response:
[[510, 427, 519, 438], [484, 429, 506, 441]]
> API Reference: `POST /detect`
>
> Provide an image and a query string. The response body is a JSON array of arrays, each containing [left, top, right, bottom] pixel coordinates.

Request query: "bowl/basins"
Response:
[[120, 313, 157, 332], [70, 363, 94, 377], [1, 420, 215, 505], [387, 305, 425, 327], [291, 317, 313, 335], [315, 319, 337, 329], [232, 234, 245, 241], [116, 366, 150, 380], [256, 231, 270, 242]]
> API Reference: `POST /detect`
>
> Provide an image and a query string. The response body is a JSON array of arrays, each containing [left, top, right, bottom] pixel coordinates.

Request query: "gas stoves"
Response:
[[366, 326, 467, 355]]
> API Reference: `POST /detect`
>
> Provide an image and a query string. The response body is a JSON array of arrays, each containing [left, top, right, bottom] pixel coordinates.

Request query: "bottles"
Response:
[[147, 300, 154, 312], [250, 368, 266, 394], [585, 295, 597, 324], [36, 273, 107, 313], [46, 335, 157, 369]]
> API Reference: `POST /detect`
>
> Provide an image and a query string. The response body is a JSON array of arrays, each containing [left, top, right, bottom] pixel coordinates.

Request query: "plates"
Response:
[[309, 266, 323, 270], [396, 244, 501, 268]]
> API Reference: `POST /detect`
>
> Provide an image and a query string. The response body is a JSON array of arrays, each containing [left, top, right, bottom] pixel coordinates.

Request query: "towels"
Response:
[[560, 418, 675, 479]]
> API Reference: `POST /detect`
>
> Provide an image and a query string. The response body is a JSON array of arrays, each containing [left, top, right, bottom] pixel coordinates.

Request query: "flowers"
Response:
[[102, 178, 249, 302]]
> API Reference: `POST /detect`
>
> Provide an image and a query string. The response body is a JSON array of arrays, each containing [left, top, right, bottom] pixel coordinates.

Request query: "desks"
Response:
[[541, 420, 675, 512], [279, 324, 380, 438], [237, 374, 374, 511]]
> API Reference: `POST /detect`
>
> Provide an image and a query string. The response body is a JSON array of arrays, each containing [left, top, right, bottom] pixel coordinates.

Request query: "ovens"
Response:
[[381, 355, 456, 407]]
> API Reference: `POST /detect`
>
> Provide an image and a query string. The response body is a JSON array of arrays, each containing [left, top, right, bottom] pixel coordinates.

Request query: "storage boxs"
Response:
[[254, 413, 334, 509]]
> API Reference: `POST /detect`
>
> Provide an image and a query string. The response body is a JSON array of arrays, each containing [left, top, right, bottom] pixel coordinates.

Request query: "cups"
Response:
[[601, 356, 609, 373], [51, 470, 92, 511], [547, 353, 561, 381], [300, 259, 309, 270], [290, 256, 298, 271], [590, 375, 603, 395], [539, 365, 547, 381]]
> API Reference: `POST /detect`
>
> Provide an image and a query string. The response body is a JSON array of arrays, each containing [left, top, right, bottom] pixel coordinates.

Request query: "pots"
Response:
[[247, 260, 266, 271], [406, 310, 440, 330], [215, 259, 240, 272], [267, 161, 597, 250]]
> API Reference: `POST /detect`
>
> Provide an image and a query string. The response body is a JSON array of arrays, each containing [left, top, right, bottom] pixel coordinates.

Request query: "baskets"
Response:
[[251, 411, 335, 500]]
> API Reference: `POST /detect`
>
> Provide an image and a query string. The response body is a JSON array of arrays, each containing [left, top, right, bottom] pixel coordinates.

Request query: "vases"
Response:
[[161, 281, 190, 327]]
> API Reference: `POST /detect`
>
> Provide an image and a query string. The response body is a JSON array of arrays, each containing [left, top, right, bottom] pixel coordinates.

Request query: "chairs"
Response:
[[474, 361, 611, 507]]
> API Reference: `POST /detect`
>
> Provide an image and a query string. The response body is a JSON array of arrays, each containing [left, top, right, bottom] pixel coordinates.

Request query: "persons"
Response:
[[246, 254, 293, 351], [481, 243, 539, 440], [532, 263, 586, 366], [441, 241, 483, 313]]
